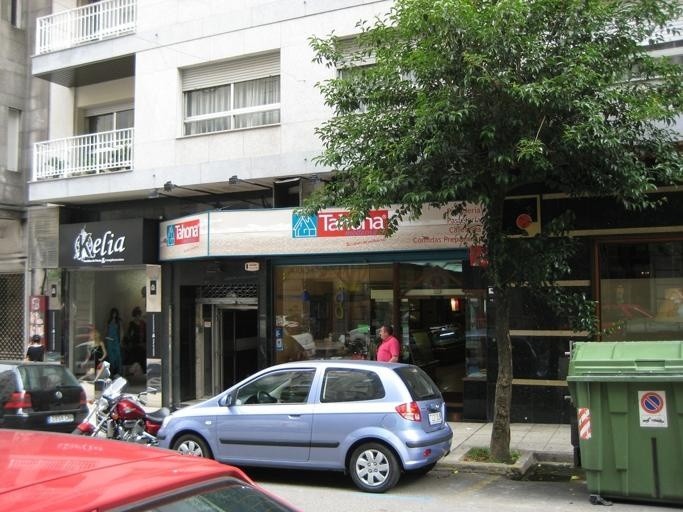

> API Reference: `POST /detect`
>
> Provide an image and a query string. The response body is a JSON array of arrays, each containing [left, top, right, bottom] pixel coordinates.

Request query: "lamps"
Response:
[[147, 188, 216, 210], [163, 181, 262, 207], [229, 176, 273, 191]]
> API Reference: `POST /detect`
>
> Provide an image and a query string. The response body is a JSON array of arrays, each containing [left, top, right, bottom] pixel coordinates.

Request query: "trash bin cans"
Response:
[[566, 341, 683, 505]]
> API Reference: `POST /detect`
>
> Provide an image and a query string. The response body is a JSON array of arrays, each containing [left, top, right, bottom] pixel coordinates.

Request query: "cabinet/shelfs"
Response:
[[426, 323, 464, 365], [486, 237, 592, 423], [462, 259, 487, 421], [401, 328, 441, 387]]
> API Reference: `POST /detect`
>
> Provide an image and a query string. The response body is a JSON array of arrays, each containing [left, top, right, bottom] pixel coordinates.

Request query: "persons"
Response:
[[126, 306, 147, 377], [277, 376, 309, 402], [25, 335, 47, 362], [79, 328, 107, 381], [375, 325, 400, 363], [101, 307, 125, 378]]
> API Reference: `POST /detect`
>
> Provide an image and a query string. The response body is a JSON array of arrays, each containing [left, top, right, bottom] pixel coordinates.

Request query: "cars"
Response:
[[156, 359, 453, 493], [0, 428, 307, 512], [0, 359, 88, 433], [342, 319, 465, 367]]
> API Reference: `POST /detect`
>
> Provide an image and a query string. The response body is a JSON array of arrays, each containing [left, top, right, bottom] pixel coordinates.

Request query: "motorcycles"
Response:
[[69, 376, 172, 446]]
